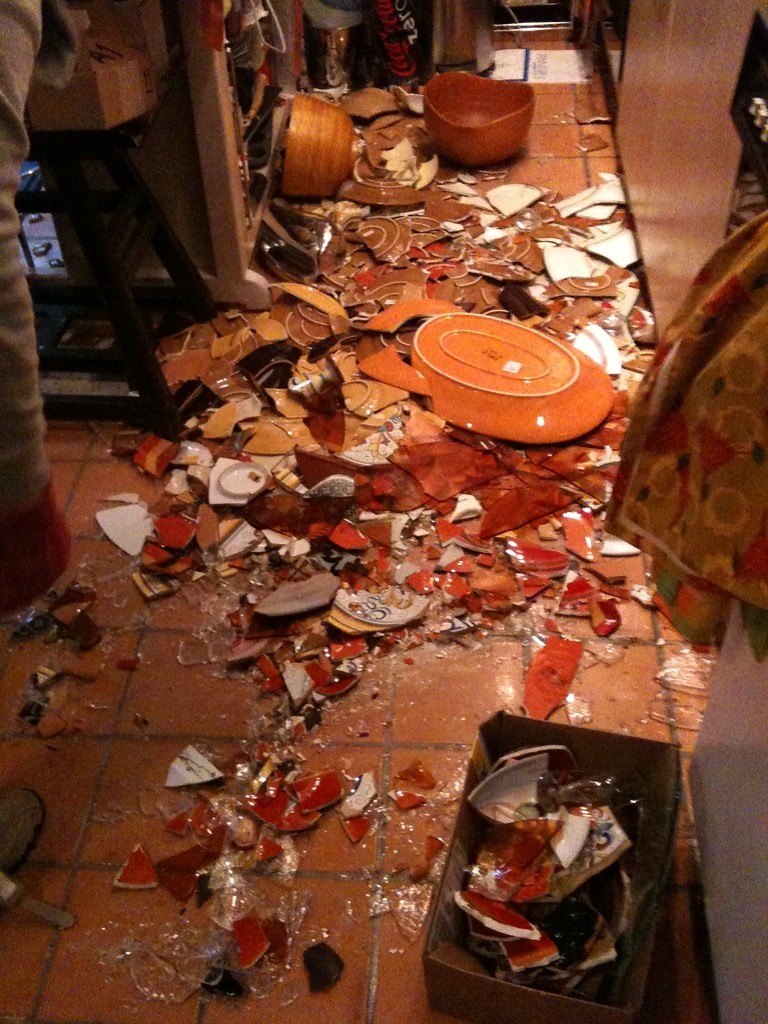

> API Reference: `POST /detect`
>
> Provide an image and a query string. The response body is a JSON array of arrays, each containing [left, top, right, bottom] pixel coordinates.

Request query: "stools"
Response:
[[15, 117, 218, 440]]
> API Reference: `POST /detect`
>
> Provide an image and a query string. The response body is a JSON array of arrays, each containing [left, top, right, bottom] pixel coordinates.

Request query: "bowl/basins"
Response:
[[424, 71, 535, 165], [281, 93, 354, 198]]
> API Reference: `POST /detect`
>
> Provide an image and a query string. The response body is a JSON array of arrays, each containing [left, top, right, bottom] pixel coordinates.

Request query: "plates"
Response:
[[410, 312, 612, 443]]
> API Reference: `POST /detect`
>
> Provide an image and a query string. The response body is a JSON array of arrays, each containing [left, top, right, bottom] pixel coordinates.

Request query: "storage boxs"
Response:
[[28, 38, 159, 130], [420, 709, 683, 1024]]
